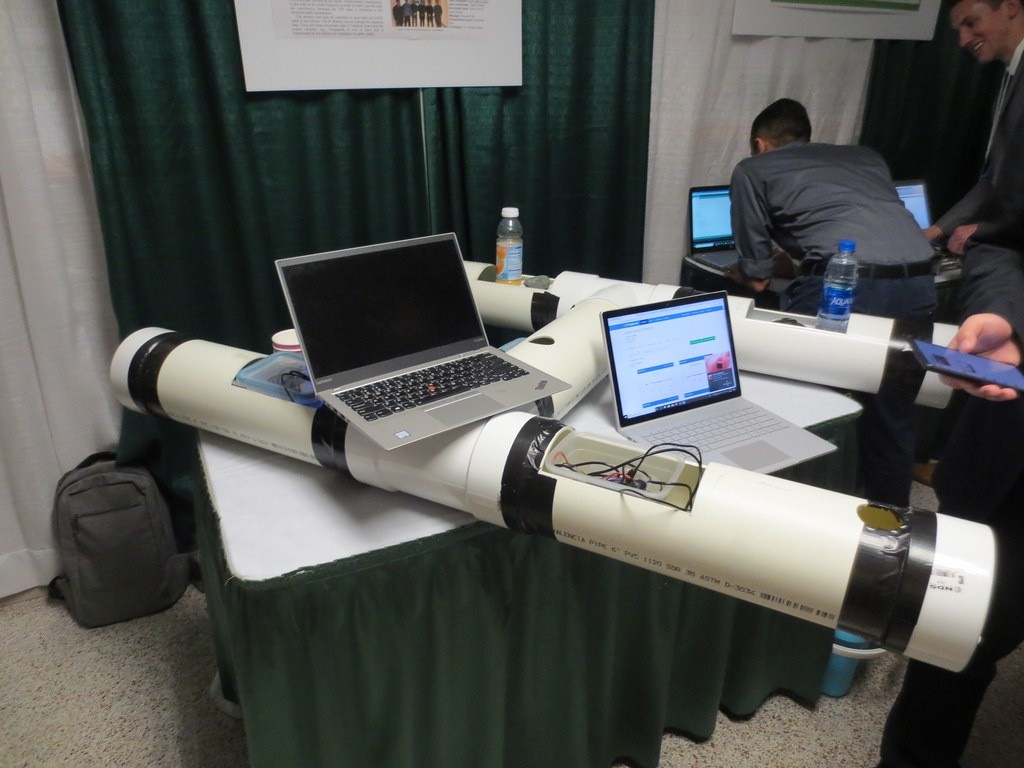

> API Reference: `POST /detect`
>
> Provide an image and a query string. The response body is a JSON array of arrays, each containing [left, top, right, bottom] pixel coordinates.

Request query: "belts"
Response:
[[798, 261, 932, 281]]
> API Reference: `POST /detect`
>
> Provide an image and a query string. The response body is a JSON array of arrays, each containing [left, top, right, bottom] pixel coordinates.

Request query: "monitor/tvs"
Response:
[[894, 178, 932, 230]]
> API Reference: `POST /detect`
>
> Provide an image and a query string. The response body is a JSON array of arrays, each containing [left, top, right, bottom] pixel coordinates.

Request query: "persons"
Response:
[[922, 0, 1024, 255], [874, 123, 1024, 768], [729, 98, 939, 512]]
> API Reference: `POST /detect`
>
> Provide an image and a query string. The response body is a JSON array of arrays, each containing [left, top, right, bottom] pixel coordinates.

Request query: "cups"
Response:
[[272, 329, 303, 357]]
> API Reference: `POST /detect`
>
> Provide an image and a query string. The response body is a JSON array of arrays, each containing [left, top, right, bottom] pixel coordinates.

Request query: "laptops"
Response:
[[275, 232, 573, 452], [686, 185, 737, 271], [600, 288, 838, 474]]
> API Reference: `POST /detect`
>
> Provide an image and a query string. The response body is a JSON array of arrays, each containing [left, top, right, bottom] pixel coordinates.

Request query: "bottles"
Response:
[[814, 240, 858, 333], [495, 207, 524, 285]]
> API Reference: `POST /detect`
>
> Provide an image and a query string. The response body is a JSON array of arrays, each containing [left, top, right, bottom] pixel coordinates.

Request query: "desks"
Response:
[[188, 371, 863, 768]]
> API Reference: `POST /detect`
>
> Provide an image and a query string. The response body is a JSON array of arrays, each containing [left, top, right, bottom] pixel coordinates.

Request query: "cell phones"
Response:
[[906, 334, 1024, 394]]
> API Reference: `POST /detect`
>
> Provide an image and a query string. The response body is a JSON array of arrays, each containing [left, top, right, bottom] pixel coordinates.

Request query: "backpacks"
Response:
[[46, 451, 189, 630]]
